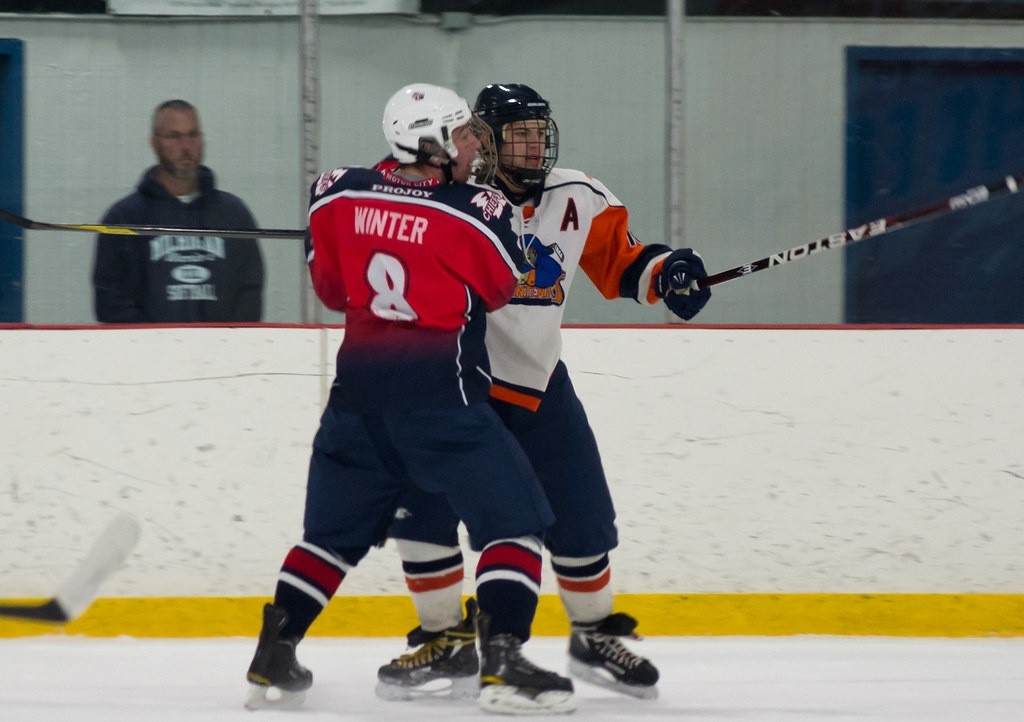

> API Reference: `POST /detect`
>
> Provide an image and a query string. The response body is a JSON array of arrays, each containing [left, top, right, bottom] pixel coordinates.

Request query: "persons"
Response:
[[246, 81, 579, 716], [92, 99, 268, 326], [375, 82, 717, 707]]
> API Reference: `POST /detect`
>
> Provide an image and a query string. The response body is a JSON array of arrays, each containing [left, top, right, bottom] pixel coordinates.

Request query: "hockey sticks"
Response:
[[1, 210, 311, 240], [0, 511, 145, 623], [670, 170, 1024, 297]]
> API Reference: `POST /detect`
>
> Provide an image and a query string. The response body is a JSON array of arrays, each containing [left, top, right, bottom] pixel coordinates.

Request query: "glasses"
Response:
[[153, 129, 205, 140]]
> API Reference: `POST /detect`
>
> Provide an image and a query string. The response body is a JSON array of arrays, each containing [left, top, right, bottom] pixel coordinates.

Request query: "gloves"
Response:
[[660, 248, 711, 321]]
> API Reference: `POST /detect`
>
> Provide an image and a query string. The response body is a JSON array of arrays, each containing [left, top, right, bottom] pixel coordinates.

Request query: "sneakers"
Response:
[[476, 638, 575, 712], [566, 629, 661, 701], [376, 601, 481, 702], [242, 602, 314, 710]]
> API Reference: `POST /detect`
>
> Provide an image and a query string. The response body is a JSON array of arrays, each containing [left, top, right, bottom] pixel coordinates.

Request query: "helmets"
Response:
[[471, 82, 560, 196], [382, 82, 474, 170]]
